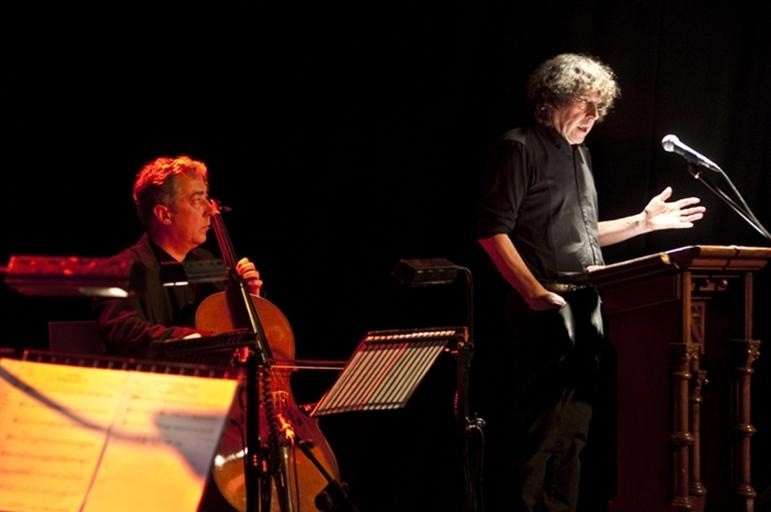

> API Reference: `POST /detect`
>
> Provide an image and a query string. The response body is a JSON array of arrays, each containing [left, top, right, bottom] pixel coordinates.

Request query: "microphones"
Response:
[[663, 132, 719, 172]]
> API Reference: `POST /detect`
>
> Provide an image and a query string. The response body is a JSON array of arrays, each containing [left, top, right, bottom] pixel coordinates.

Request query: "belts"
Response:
[[576, 284, 592, 289]]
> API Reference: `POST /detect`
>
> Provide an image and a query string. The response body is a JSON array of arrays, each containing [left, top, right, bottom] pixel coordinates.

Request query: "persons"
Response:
[[95, 156, 263, 365], [477, 53, 708, 512]]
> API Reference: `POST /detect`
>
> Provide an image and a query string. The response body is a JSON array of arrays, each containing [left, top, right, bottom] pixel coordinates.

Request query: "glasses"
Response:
[[575, 98, 603, 112]]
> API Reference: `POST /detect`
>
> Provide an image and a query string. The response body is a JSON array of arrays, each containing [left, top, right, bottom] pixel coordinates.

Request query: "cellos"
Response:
[[179, 197, 342, 512]]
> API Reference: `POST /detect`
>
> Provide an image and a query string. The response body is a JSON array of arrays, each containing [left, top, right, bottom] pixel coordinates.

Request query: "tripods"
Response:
[[244, 377, 294, 510]]
